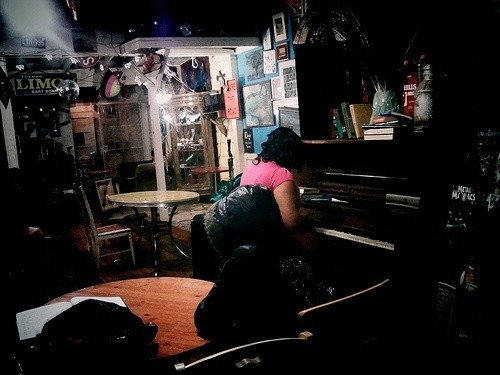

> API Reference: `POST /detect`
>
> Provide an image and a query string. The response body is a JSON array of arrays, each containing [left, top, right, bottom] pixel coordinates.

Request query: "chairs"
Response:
[[175, 278, 391, 374], [73, 177, 147, 271]]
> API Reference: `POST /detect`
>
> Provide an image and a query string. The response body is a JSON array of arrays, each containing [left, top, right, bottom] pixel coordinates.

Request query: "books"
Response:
[[16, 296, 127, 340], [299, 186, 323, 200], [384, 193, 424, 211], [333, 102, 394, 141]]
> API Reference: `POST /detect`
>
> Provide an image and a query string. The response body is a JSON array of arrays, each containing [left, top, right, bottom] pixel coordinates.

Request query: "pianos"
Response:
[[292, 137, 436, 313]]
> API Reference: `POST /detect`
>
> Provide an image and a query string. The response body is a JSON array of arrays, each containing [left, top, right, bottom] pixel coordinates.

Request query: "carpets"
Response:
[[74, 222, 195, 281]]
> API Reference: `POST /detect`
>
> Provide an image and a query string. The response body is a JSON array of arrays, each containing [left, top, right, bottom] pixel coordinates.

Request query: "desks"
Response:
[[40, 276, 215, 360], [106, 190, 200, 276], [190, 167, 229, 194]]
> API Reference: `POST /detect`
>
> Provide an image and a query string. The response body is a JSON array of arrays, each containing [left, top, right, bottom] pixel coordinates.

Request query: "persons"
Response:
[[206, 127, 334, 278]]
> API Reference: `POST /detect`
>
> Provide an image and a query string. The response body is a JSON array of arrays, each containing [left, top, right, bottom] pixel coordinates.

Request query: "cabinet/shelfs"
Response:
[[96, 102, 150, 171], [169, 92, 215, 195]]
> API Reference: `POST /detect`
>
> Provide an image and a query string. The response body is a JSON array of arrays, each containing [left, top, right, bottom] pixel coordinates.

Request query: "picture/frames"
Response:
[[275, 42, 290, 62], [273, 12, 287, 42]]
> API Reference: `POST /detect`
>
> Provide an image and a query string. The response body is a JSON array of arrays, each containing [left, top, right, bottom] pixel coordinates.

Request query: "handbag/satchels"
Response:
[[26, 298, 160, 370]]
